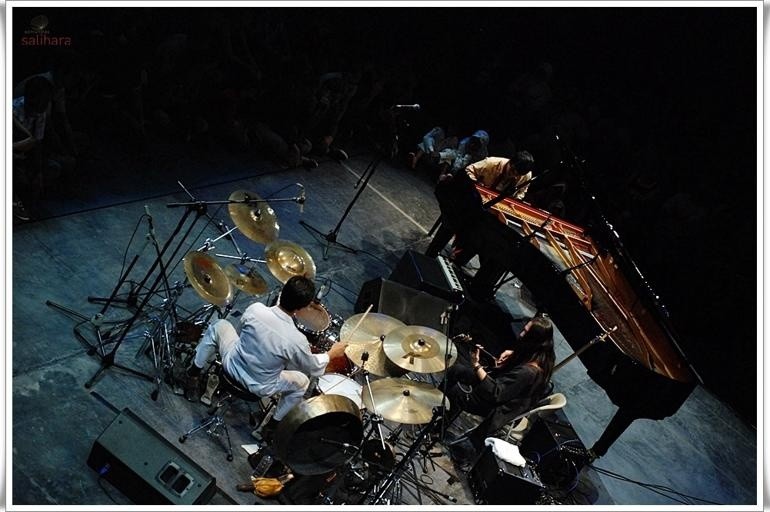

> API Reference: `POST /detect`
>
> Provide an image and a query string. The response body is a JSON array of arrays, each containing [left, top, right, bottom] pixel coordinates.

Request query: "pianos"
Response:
[[425, 176, 704, 465]]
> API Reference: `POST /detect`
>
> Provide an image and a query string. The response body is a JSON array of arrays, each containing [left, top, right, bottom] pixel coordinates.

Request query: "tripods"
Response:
[[299, 109, 410, 260], [384, 424, 436, 505], [45, 181, 306, 400]]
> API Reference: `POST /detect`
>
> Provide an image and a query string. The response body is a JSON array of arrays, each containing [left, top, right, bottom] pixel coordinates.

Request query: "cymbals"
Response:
[[184, 251, 234, 306], [340, 313, 408, 377], [265, 239, 317, 285], [383, 325, 458, 373], [362, 378, 451, 424], [362, 439, 396, 470], [224, 264, 266, 293], [275, 394, 364, 477], [228, 190, 280, 245]]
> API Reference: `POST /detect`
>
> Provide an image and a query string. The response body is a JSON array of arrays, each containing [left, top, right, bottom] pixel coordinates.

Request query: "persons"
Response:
[[170, 275, 352, 445], [405, 315, 556, 446], [12, 9, 756, 261]]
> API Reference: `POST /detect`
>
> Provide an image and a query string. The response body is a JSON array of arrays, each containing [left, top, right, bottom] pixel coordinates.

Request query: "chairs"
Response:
[[448, 392, 567, 446]]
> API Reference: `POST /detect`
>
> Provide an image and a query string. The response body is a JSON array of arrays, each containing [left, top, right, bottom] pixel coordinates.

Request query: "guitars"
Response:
[[453, 334, 502, 372]]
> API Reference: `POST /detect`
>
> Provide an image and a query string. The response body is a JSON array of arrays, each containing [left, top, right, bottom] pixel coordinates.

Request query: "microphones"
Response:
[[395, 104, 420, 109], [313, 282, 328, 306]]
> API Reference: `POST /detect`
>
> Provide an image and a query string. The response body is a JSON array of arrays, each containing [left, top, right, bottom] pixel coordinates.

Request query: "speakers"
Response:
[[353, 276, 454, 337], [87, 407, 217, 505]]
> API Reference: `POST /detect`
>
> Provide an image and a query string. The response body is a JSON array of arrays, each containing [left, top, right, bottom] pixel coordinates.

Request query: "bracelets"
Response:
[[472, 364, 483, 373]]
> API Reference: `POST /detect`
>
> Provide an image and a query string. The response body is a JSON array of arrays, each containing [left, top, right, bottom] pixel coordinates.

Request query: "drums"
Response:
[[292, 300, 355, 379], [310, 372, 367, 413]]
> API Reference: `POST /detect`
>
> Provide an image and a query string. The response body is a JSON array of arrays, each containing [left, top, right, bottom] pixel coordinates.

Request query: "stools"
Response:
[[180, 366, 262, 461]]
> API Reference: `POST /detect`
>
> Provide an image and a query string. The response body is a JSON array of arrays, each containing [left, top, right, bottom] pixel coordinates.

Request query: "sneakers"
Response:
[[261, 424, 273, 441], [172, 367, 199, 391], [405, 428, 437, 442], [407, 151, 416, 169], [439, 173, 452, 182]]
